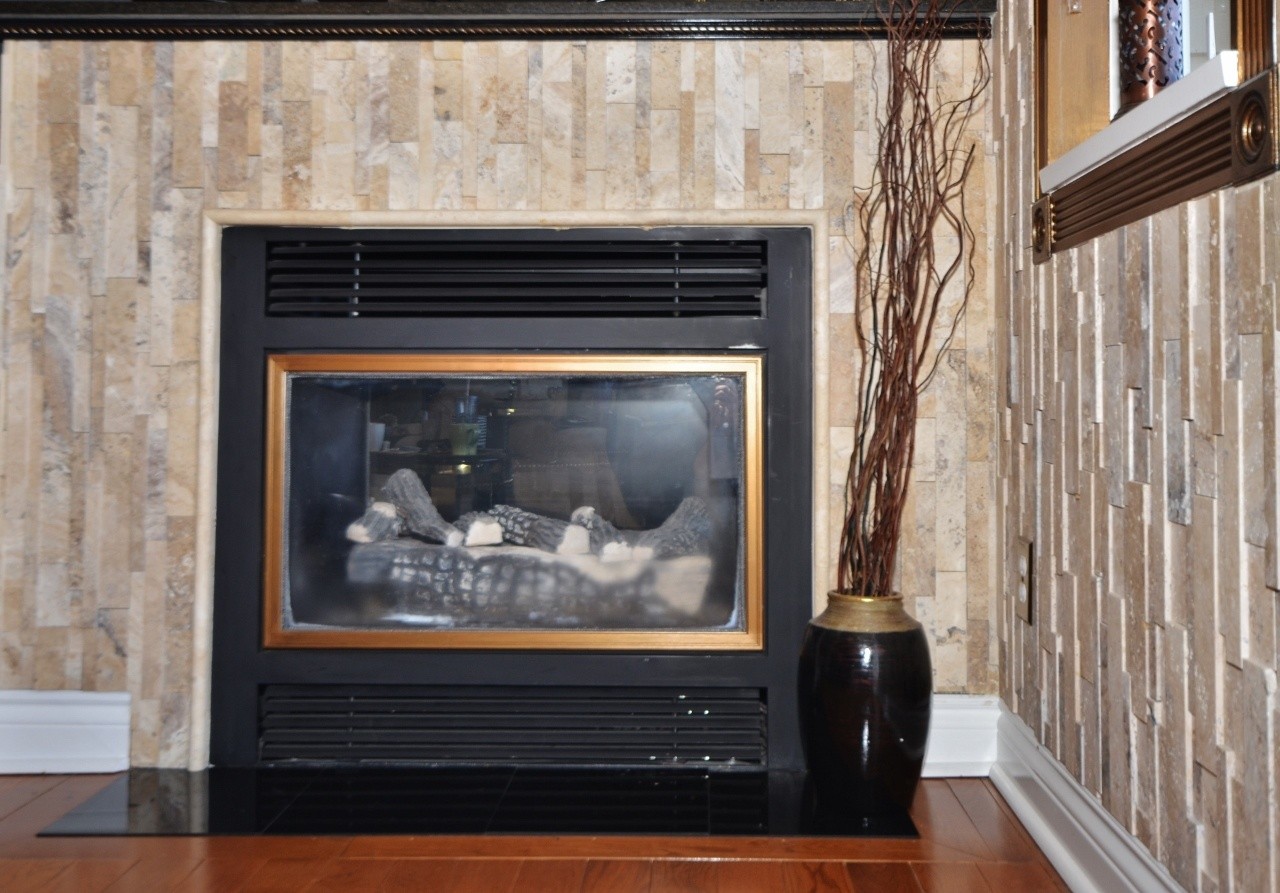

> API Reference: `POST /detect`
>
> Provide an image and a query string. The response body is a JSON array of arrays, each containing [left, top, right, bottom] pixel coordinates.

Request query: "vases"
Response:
[[796, 590, 934, 829]]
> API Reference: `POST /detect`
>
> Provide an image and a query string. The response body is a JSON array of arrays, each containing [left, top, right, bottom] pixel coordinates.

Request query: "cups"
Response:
[[368, 422, 385, 451], [450, 423, 482, 456]]
